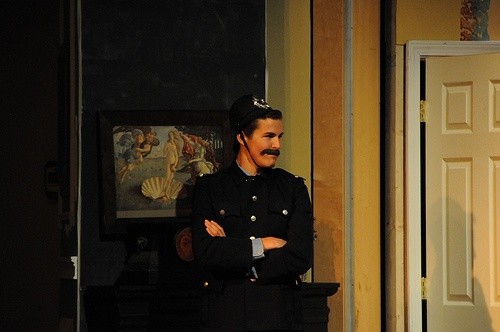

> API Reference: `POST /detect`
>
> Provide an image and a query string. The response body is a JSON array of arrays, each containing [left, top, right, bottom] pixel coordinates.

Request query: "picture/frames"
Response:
[[97, 109, 236, 236]]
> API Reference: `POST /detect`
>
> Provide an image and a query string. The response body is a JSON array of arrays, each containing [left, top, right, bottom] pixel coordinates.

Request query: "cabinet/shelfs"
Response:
[[84, 284, 339, 332]]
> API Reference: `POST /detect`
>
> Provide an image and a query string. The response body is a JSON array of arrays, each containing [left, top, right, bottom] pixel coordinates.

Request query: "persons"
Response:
[[191, 94, 314, 332]]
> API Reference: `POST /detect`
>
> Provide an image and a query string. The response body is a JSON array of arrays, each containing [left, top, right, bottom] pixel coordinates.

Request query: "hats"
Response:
[[229, 94, 273, 134]]
